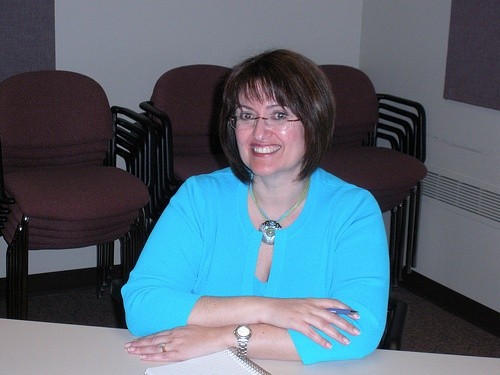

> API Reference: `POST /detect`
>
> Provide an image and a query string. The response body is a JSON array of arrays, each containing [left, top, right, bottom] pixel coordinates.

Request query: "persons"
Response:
[[121, 48, 391, 367]]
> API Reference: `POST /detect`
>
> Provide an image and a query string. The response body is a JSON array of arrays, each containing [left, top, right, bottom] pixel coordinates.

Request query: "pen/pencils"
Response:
[[327, 307, 359, 316]]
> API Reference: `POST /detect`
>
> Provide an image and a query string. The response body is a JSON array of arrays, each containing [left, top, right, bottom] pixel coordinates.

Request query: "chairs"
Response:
[[0, 64, 428, 321]]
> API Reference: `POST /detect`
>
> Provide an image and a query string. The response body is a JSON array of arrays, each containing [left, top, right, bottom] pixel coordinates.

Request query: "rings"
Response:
[[159, 343, 169, 353]]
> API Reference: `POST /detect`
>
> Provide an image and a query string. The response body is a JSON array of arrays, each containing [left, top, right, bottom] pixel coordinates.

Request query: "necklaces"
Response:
[[247, 173, 309, 246]]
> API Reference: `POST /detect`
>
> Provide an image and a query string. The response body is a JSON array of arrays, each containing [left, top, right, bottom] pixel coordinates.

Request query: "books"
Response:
[[145, 348, 272, 375]]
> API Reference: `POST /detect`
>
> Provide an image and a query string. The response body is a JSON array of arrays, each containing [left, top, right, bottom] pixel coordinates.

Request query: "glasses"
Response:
[[229, 112, 300, 131]]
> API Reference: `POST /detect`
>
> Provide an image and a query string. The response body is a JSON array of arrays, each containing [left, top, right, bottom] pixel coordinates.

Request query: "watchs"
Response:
[[232, 326, 252, 358]]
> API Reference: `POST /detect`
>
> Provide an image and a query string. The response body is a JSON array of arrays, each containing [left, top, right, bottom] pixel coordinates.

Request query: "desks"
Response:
[[0, 318, 500, 375]]
